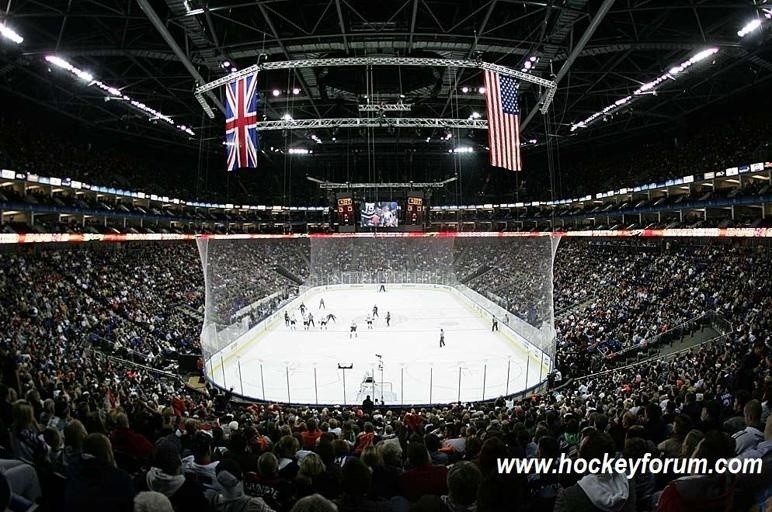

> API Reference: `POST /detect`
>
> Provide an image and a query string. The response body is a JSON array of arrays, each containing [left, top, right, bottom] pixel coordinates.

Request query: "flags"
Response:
[[482, 69, 522, 172], [224, 72, 257, 171]]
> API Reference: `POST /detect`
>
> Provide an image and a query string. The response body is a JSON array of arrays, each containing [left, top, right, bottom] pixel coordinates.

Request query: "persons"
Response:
[[319, 299, 326, 309], [308, 395, 466, 512], [466, 283, 772, 512], [374, 351, 383, 371], [365, 314, 375, 329], [327, 314, 336, 323], [348, 320, 358, 340], [439, 328, 446, 347], [309, 313, 315, 328], [1, 284, 308, 512], [386, 312, 391, 327], [372, 304, 379, 319], [319, 315, 328, 330], [0, 179, 772, 284], [380, 280, 386, 292]]
